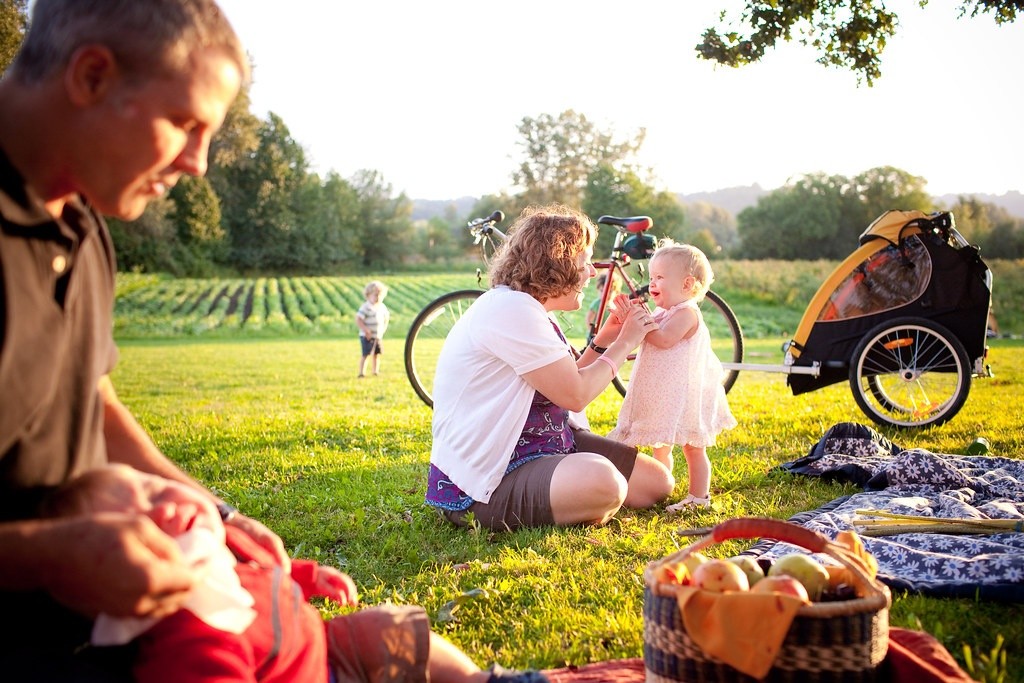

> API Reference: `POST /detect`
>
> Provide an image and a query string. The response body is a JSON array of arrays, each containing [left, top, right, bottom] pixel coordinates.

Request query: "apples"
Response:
[[653, 531, 877, 600]]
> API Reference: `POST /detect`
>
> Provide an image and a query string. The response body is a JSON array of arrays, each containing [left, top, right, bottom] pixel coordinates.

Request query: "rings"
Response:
[[643, 317, 647, 323]]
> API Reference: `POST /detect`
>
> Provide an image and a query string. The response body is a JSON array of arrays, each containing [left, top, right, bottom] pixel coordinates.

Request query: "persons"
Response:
[[607, 237, 739, 516], [42, 464, 550, 683], [355, 281, 391, 378], [0, 0, 294, 683], [424, 202, 675, 534]]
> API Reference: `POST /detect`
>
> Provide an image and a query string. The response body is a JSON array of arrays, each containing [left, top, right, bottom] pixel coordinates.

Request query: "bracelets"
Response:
[[598, 356, 618, 378], [589, 338, 607, 354], [217, 504, 235, 521]]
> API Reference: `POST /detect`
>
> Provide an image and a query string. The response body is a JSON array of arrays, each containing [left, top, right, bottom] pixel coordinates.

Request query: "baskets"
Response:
[[643, 518, 892, 683]]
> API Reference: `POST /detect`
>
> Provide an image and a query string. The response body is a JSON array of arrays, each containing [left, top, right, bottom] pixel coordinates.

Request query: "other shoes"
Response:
[[486, 660, 547, 683]]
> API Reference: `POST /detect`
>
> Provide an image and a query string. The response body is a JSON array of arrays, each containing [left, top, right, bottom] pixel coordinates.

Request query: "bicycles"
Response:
[[402, 211, 743, 414]]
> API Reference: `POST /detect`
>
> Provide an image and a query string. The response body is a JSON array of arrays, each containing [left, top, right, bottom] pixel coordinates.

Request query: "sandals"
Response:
[[665, 492, 712, 516]]
